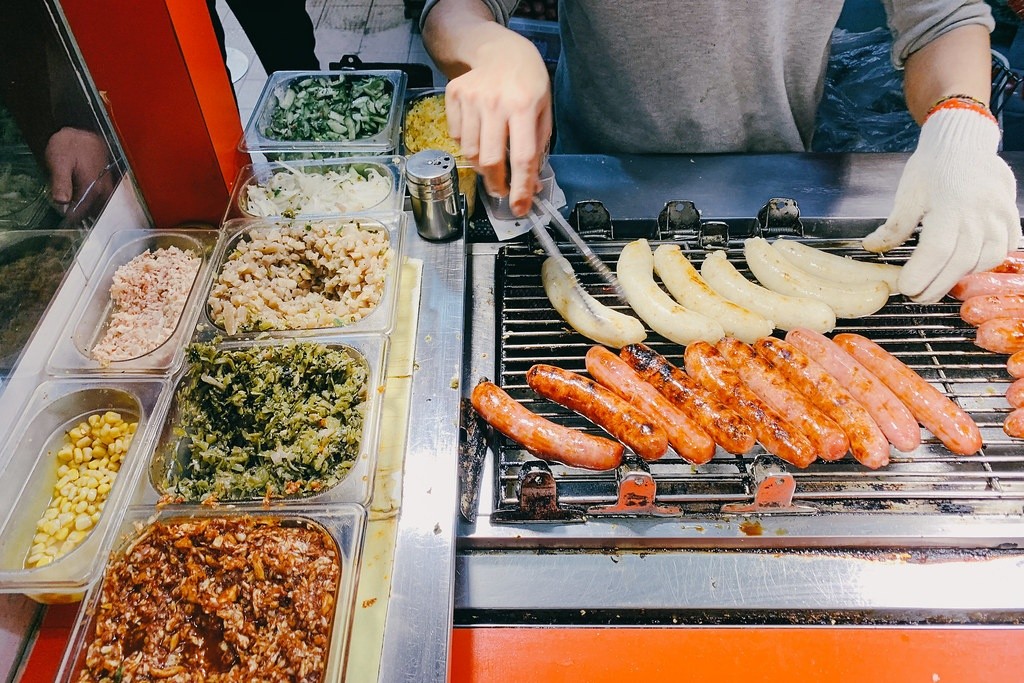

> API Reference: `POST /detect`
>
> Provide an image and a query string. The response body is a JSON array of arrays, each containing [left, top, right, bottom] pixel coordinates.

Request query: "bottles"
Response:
[[405, 149, 463, 242]]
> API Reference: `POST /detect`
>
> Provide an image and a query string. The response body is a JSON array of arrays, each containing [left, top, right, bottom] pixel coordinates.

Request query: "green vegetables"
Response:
[[155, 334, 367, 500]]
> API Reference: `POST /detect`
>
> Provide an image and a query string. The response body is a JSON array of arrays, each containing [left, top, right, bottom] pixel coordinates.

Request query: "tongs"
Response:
[[501, 147, 629, 323]]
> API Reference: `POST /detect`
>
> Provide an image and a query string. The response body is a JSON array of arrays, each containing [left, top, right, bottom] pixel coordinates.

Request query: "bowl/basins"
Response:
[[0, 71, 407, 683]]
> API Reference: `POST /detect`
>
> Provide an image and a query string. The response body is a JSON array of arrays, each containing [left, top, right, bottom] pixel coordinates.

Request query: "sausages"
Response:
[[470, 237, 1024, 471]]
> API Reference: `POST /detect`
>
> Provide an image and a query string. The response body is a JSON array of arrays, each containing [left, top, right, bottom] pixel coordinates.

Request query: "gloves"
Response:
[[861, 100, 1022, 304]]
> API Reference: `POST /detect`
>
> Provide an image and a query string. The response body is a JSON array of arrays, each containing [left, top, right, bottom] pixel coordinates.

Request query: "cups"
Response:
[[404, 87, 474, 217]]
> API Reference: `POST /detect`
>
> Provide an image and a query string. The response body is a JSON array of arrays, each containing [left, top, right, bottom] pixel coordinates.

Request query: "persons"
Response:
[[0, 0, 114, 225], [205, 1, 319, 115], [406, 0, 1023, 303]]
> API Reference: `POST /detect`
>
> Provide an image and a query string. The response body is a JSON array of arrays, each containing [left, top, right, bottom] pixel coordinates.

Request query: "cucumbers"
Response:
[[265, 75, 391, 172]]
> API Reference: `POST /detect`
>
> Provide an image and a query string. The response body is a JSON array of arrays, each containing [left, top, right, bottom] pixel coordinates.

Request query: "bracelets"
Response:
[[925, 94, 990, 113]]
